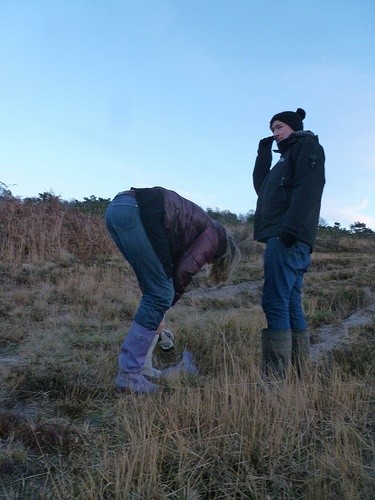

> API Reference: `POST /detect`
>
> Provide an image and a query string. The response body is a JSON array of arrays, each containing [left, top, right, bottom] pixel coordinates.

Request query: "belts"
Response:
[[113, 191, 136, 200]]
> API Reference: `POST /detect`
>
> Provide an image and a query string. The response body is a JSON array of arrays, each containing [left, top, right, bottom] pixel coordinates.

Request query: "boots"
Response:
[[115, 320, 163, 394], [163, 351, 197, 385], [292, 329, 313, 369], [262, 329, 292, 374]]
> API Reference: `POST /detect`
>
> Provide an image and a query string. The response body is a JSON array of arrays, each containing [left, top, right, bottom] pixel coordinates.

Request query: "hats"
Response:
[[270, 108, 306, 131]]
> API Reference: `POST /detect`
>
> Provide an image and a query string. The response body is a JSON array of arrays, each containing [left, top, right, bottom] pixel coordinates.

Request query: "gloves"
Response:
[[280, 231, 297, 248], [258, 136, 276, 155]]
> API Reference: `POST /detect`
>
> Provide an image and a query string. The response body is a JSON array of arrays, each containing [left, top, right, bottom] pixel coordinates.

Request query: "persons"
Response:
[[105, 186, 241, 394], [253, 108, 326, 377]]
